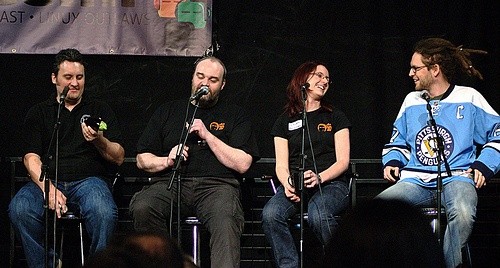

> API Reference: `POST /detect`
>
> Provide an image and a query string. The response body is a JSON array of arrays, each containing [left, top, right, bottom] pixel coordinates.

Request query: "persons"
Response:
[[262, 62, 351, 268], [374, 38, 500, 268], [7, 48, 127, 268], [128, 56, 262, 268]]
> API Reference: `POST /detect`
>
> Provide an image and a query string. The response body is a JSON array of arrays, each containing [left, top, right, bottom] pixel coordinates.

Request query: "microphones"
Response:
[[301, 83, 310, 90], [420, 89, 431, 102], [60, 86, 70, 101], [189, 86, 209, 102]]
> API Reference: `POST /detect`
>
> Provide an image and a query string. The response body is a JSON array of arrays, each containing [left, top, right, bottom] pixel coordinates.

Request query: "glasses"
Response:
[[409, 65, 428, 73], [308, 72, 331, 83]]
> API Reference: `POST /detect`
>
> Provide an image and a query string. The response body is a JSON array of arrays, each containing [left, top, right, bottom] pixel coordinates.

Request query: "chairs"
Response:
[[184, 176, 247, 268], [422, 206, 472, 268], [262, 172, 358, 268], [49, 172, 122, 268]]
